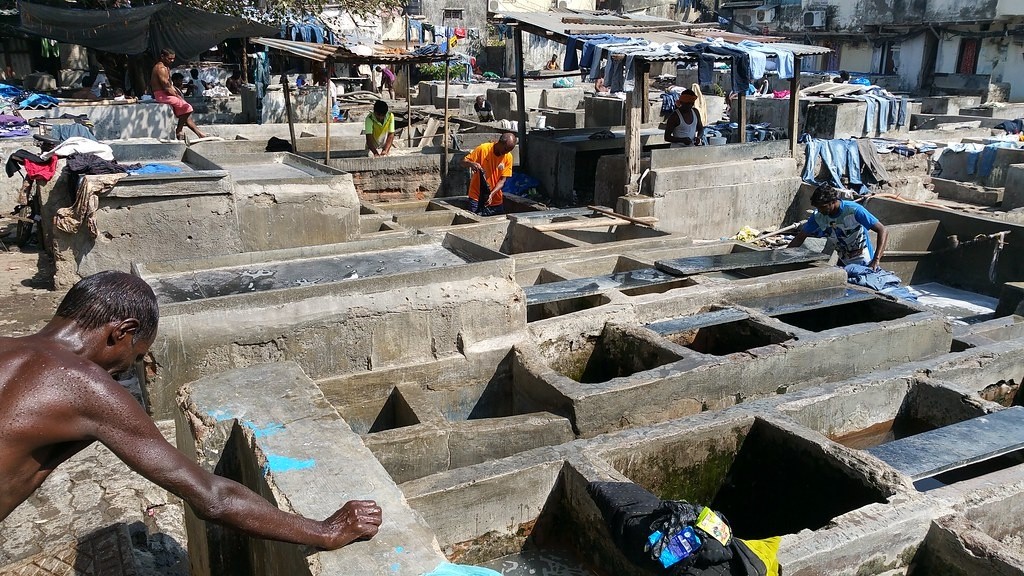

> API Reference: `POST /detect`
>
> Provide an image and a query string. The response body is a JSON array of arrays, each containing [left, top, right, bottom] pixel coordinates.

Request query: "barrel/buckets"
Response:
[[709, 137, 727, 145]]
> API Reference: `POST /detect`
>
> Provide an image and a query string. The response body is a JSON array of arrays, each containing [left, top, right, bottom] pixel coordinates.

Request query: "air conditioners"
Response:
[[557, 0, 570, 9], [488, 0, 503, 12], [755, 9, 775, 24], [803, 11, 826, 28]]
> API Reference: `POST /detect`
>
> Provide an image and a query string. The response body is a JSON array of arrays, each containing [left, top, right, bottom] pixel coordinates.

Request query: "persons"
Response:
[[71, 76, 97, 100], [98, 88, 114, 100], [376, 67, 395, 101], [183, 69, 212, 98], [595, 67, 610, 93], [746, 77, 760, 96], [324, 76, 337, 105], [364, 100, 396, 158], [460, 132, 517, 217], [296, 74, 309, 88], [727, 88, 737, 111], [834, 70, 852, 84], [0, 269, 383, 551], [153, 47, 207, 140], [474, 95, 497, 123], [107, 87, 134, 99], [786, 185, 890, 272], [546, 54, 561, 70], [664, 88, 705, 148], [171, 73, 198, 126]]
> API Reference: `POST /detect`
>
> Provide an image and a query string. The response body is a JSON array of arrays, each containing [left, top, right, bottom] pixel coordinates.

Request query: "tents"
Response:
[[250, 33, 462, 182], [12, 0, 283, 67], [497, 8, 836, 197]]
